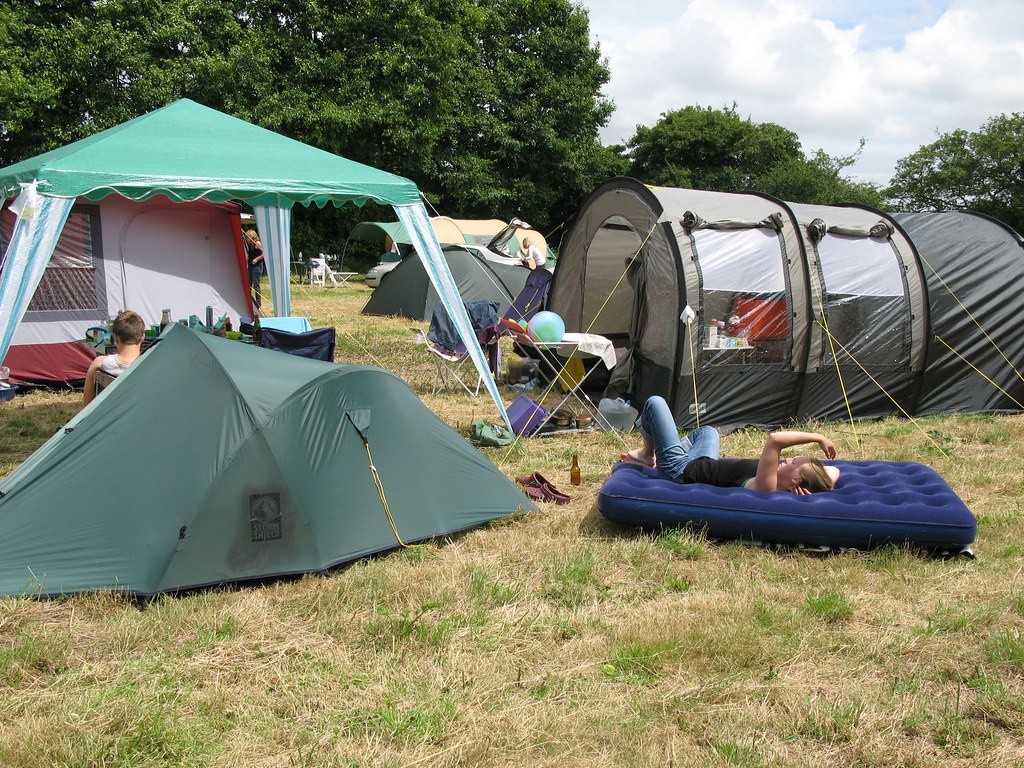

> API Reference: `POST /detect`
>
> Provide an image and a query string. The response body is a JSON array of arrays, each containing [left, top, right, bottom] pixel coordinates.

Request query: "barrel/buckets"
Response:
[[508, 358, 541, 385], [595, 397, 639, 434]]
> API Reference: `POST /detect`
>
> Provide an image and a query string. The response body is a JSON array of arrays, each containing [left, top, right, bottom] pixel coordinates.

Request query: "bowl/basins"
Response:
[[0, 384, 19, 403], [226, 331, 242, 340]]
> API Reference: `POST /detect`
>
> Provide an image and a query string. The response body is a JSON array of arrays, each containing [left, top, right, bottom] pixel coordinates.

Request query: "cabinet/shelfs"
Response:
[[307, 258, 325, 286]]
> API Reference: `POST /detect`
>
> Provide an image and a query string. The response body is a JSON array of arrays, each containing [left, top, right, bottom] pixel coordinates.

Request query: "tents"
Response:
[[341, 217, 508, 273], [0, 98, 519, 441], [0, 321, 550, 598], [360, 244, 532, 321], [498, 269, 553, 333], [487, 217, 557, 269], [543, 178, 1024, 430]]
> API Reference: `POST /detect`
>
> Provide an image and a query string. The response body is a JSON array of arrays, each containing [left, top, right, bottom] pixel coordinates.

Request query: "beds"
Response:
[[599, 458, 977, 547]]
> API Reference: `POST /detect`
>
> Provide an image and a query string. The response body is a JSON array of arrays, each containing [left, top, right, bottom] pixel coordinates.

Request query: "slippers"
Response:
[[516, 472, 556, 489], [526, 483, 570, 505]]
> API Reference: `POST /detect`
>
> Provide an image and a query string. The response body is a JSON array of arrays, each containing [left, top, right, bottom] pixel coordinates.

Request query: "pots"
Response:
[[530, 408, 596, 435]]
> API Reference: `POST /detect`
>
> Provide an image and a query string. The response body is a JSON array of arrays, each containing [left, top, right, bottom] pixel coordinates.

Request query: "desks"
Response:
[[330, 272, 358, 289], [507, 328, 621, 440], [703, 344, 754, 364], [291, 261, 309, 284]]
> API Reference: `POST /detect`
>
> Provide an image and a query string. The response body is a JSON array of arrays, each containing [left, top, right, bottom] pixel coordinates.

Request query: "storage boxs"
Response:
[[510, 359, 541, 388], [499, 396, 547, 437]]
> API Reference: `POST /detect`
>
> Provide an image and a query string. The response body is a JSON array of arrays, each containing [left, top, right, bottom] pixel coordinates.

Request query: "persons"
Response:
[[619, 395, 840, 494], [519, 237, 546, 269], [83, 311, 145, 408], [245, 229, 264, 307]]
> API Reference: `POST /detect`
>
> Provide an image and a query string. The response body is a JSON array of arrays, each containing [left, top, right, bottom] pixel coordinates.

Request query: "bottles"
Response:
[[298, 252, 303, 262], [160, 308, 173, 333], [252, 315, 261, 341], [225, 317, 232, 338], [571, 455, 581, 486], [703, 318, 749, 348], [206, 305, 213, 330], [179, 319, 188, 328]]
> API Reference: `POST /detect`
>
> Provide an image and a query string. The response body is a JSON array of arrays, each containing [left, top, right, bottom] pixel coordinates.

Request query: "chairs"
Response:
[[410, 302, 503, 398], [254, 325, 337, 363]]
